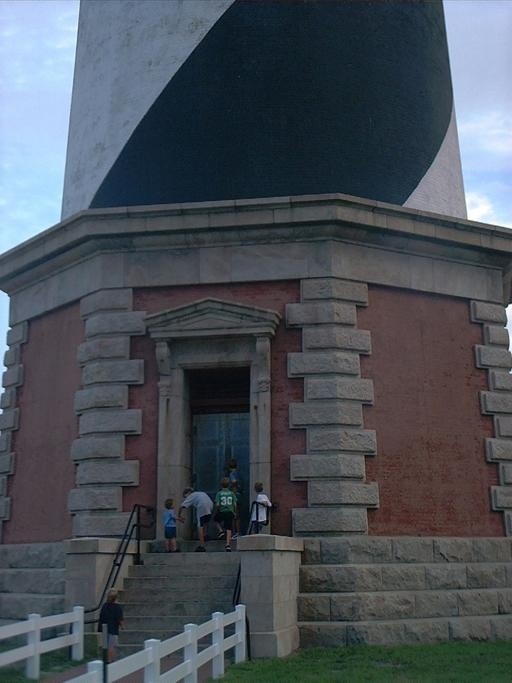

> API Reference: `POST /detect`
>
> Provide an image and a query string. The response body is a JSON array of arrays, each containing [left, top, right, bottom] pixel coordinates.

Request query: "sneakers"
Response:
[[195, 546, 205, 551], [216, 531, 225, 539], [225, 545, 231, 551], [231, 532, 239, 539]]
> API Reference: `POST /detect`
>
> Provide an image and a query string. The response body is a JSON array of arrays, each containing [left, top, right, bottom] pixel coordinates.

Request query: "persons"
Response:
[[178, 486, 213, 551], [227, 457, 244, 540], [214, 477, 239, 551], [250, 480, 273, 534], [98, 587, 127, 663], [165, 497, 185, 552]]
